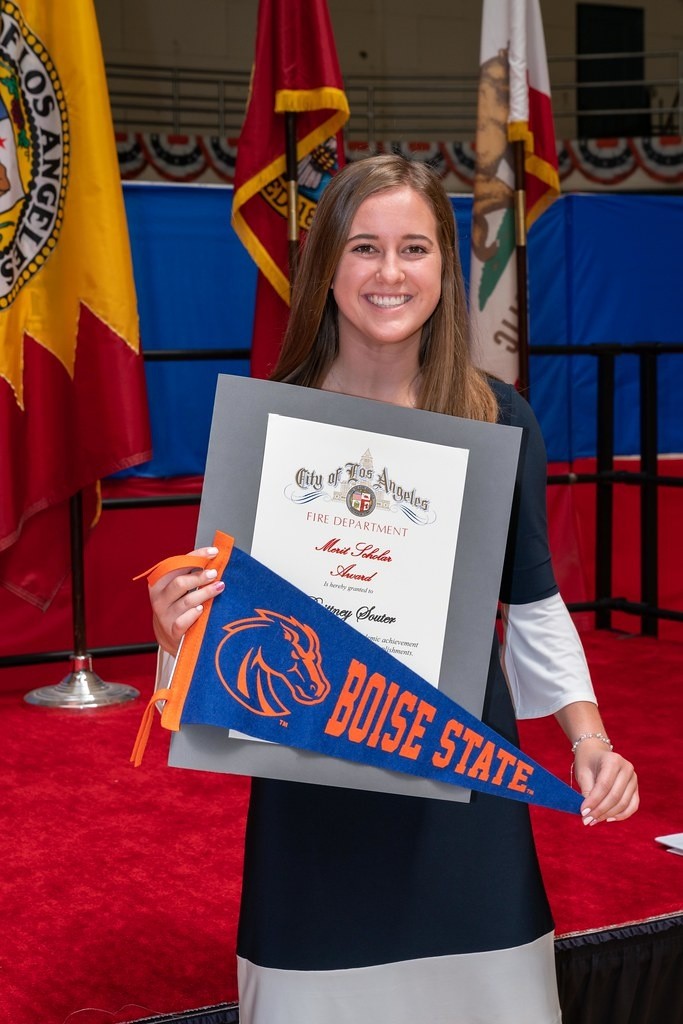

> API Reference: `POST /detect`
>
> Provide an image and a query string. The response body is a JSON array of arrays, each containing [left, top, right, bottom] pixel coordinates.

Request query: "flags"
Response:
[[472, 1, 562, 392], [0, 0, 155, 612], [226, 0, 350, 384]]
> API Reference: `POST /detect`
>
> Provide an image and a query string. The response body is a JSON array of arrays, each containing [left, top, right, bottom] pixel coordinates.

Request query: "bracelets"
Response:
[[570, 731, 614, 790]]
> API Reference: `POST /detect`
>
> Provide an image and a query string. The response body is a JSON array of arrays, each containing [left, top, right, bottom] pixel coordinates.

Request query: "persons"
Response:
[[144, 153, 643, 1024]]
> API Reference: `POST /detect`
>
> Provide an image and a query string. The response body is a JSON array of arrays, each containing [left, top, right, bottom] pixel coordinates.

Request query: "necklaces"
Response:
[[331, 366, 422, 410]]
